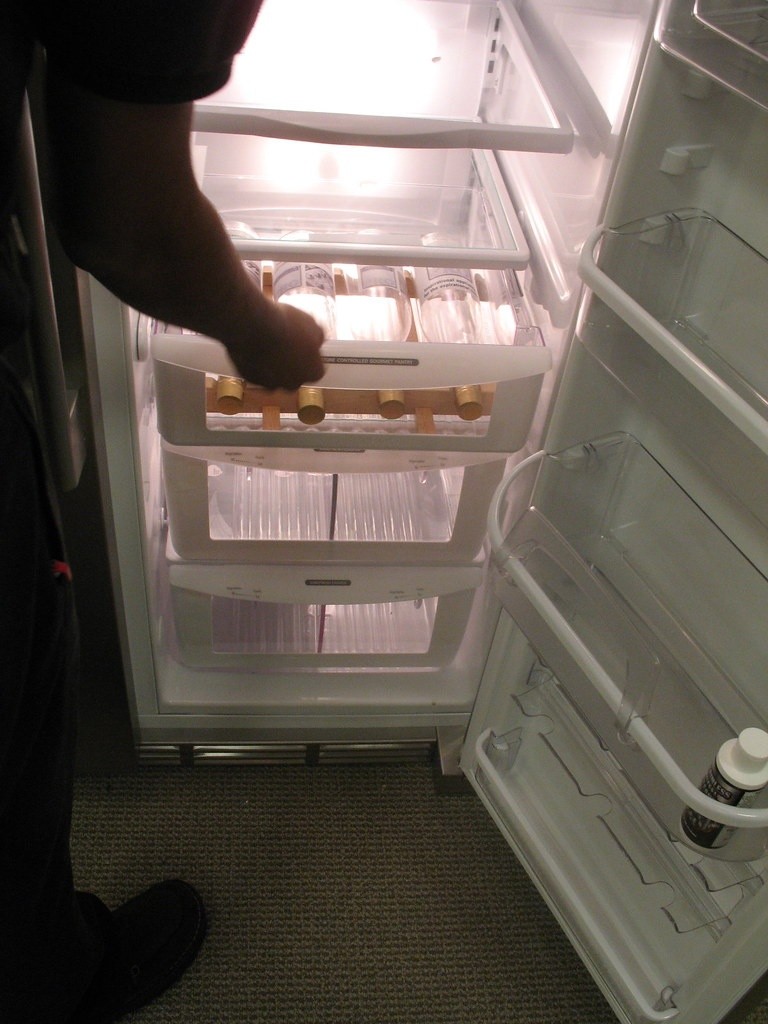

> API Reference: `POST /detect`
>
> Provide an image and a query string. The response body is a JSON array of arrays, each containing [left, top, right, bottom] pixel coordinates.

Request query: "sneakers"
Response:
[[83, 879, 207, 1023]]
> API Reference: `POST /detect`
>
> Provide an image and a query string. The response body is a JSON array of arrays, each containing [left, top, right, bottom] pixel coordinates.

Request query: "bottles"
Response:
[[341, 233, 413, 419], [406, 233, 484, 421], [679, 727, 767, 852], [272, 229, 339, 424], [217, 221, 263, 416]]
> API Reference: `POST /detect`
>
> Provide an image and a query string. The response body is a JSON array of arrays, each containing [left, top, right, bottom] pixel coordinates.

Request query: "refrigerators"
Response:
[[73, 4, 768, 1024]]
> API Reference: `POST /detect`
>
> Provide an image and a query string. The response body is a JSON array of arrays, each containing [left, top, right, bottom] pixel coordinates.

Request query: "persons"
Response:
[[0, 0, 326, 1023]]
